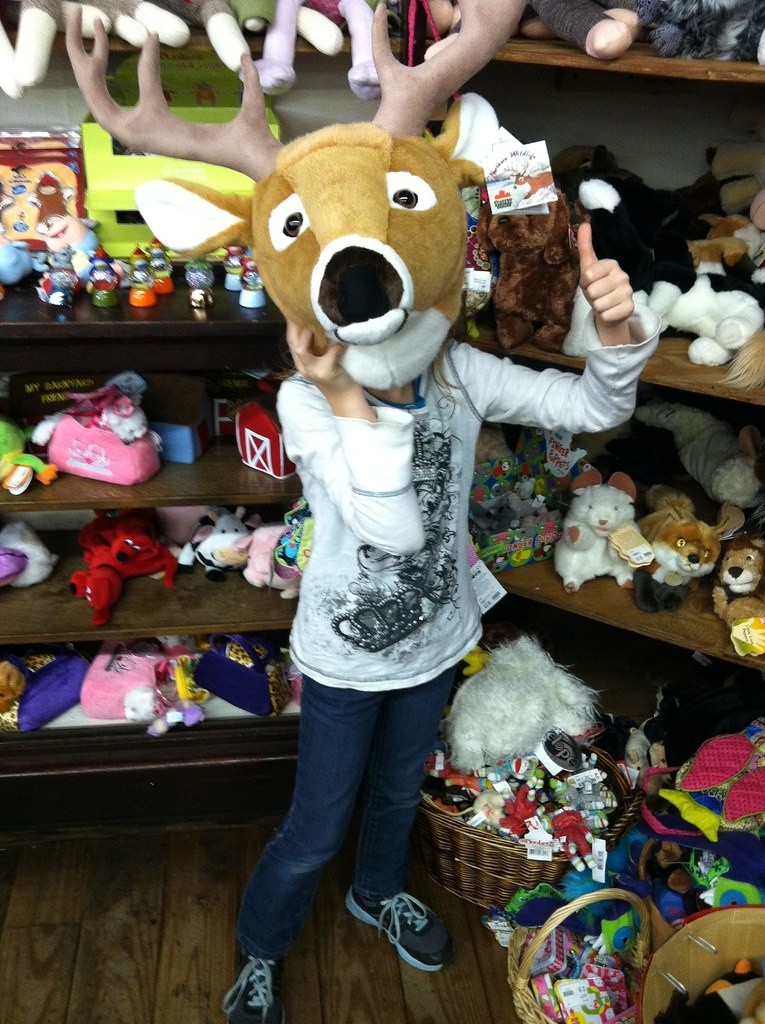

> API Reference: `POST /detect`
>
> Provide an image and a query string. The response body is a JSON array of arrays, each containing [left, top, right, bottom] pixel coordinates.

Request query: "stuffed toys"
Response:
[[0, 0, 765, 1024]]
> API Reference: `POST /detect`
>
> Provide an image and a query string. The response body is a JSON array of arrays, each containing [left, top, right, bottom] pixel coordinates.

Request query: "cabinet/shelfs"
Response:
[[1, 31, 401, 833], [465, 44, 765, 725]]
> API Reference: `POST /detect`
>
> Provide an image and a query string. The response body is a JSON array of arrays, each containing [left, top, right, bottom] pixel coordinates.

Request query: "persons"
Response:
[[216, 223, 660, 1023]]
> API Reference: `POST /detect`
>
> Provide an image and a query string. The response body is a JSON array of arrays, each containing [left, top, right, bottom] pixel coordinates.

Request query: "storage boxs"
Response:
[[638, 903, 765, 1024]]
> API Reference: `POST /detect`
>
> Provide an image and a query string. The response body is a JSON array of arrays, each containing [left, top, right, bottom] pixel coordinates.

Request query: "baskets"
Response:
[[416, 742, 643, 909], [508, 889, 653, 1024]]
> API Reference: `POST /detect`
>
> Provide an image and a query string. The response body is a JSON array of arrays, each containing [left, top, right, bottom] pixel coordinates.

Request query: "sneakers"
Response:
[[220, 935, 285, 1024], [345, 884, 449, 971]]
